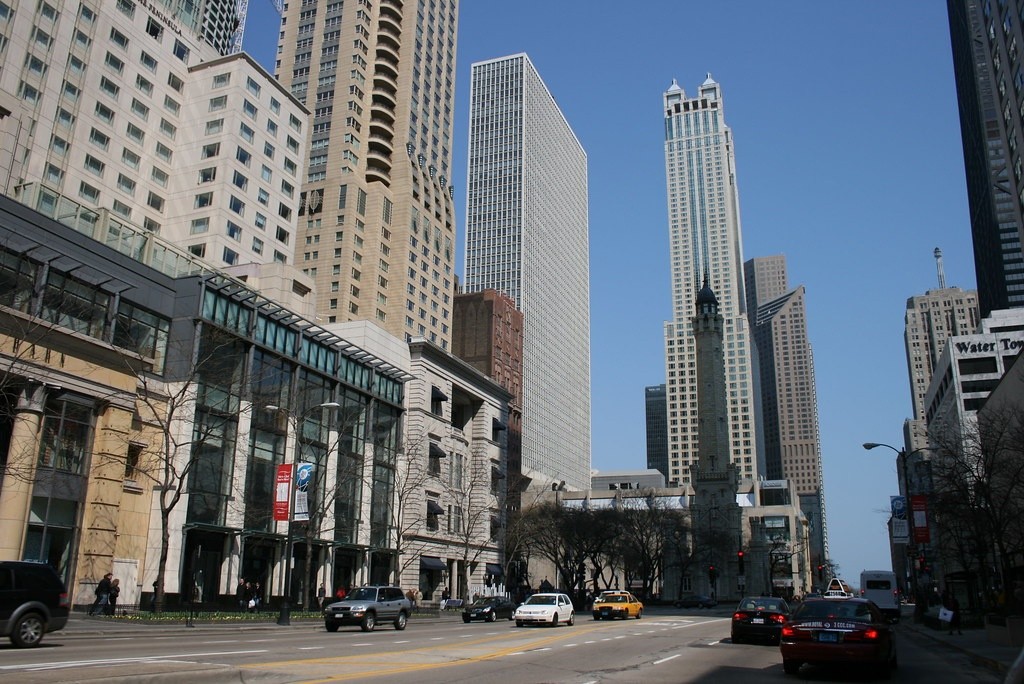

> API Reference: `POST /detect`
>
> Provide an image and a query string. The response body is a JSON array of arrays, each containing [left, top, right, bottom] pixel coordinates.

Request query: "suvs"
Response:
[[323, 583, 413, 632], [0, 559, 71, 649]]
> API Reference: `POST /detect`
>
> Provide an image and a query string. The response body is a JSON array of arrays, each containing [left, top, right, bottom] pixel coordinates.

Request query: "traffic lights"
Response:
[[739, 551, 743, 561], [818, 566, 822, 575], [709, 566, 713, 576]]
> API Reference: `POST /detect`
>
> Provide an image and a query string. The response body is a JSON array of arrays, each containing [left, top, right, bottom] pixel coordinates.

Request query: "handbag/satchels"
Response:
[[938, 606, 954, 622], [248, 599, 256, 608]]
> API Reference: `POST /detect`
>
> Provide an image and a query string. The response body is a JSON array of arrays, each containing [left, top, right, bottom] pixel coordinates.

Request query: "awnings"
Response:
[[494, 419, 506, 430], [491, 517, 505, 528], [487, 564, 504, 575], [420, 557, 447, 570], [432, 387, 448, 401], [430, 443, 446, 457], [428, 501, 444, 515], [492, 468, 505, 479]]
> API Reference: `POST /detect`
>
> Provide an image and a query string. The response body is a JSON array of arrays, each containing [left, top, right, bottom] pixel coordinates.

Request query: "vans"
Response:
[[860, 569, 902, 620]]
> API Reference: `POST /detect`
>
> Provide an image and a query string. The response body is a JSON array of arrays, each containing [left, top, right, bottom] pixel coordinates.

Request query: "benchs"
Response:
[[443, 599, 463, 611]]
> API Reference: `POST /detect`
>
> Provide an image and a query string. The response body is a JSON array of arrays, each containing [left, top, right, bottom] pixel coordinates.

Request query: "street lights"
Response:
[[862, 441, 938, 607], [266, 402, 340, 625]]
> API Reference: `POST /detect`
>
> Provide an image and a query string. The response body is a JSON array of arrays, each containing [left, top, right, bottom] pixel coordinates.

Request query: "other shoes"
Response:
[[958, 630, 963, 635], [948, 631, 953, 636]]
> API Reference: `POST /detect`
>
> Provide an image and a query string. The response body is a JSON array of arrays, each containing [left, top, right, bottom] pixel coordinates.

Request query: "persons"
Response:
[[337, 585, 345, 599], [150, 575, 159, 611], [236, 578, 262, 613], [406, 589, 423, 613], [516, 591, 522, 603], [318, 583, 326, 607], [89, 573, 120, 615], [711, 592, 714, 599], [933, 590, 962, 635], [587, 595, 592, 611], [987, 585, 1024, 617], [787, 586, 794, 601], [194, 570, 203, 601], [442, 587, 451, 602]]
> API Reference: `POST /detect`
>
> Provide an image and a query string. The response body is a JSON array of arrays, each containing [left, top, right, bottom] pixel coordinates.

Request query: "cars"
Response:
[[731, 596, 791, 645], [462, 596, 520, 623], [592, 591, 643, 619], [515, 592, 575, 628], [801, 578, 855, 602], [779, 597, 900, 681]]
